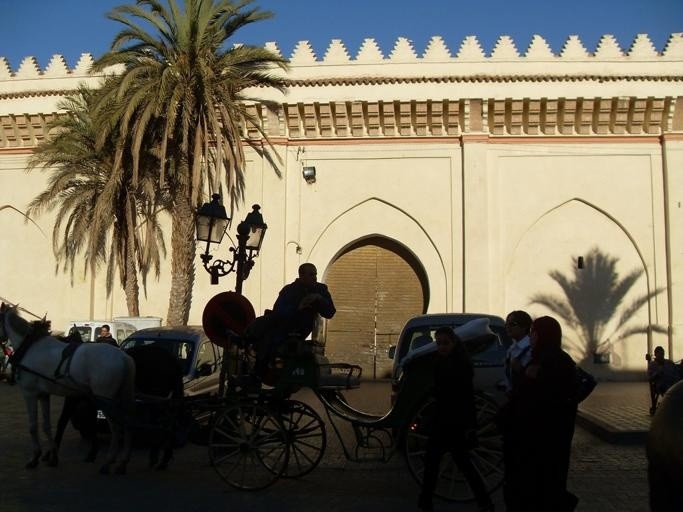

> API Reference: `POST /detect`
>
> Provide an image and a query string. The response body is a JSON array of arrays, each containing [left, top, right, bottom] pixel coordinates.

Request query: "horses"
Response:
[[0, 302, 136, 475]]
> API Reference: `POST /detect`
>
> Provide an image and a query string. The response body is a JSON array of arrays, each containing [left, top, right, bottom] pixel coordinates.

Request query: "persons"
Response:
[[96, 324, 117, 347], [505, 314, 580, 511], [648, 345, 675, 376], [267, 262, 338, 369], [415, 327, 498, 511], [501, 309, 595, 411]]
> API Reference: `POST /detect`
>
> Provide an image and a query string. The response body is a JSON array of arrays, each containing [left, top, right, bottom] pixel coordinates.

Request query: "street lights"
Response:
[[283, 239, 300, 290], [192, 194, 267, 422]]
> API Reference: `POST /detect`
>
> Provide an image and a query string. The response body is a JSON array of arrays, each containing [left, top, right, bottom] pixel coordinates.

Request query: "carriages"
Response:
[[0, 302, 517, 501]]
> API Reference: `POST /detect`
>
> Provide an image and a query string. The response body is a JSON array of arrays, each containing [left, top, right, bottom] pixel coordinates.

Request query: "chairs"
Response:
[[303, 340, 362, 390], [646, 353, 683, 414], [411, 335, 434, 350]]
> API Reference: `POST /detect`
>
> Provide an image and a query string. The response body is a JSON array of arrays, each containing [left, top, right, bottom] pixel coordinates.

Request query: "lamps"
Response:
[[303, 167, 315, 184]]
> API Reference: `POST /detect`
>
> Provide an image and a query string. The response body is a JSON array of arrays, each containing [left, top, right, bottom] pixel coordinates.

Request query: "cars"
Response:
[[387, 315, 508, 407]]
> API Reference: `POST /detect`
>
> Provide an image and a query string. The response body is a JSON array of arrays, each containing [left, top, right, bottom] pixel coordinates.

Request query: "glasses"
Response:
[[506, 320, 518, 327], [435, 340, 451, 345], [529, 327, 535, 334]]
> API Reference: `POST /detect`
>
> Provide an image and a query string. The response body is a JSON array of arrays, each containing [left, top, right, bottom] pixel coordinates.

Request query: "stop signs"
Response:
[[201, 292, 255, 349]]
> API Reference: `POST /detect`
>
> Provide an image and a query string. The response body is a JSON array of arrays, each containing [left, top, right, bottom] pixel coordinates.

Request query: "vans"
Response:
[[65, 315, 229, 444]]
